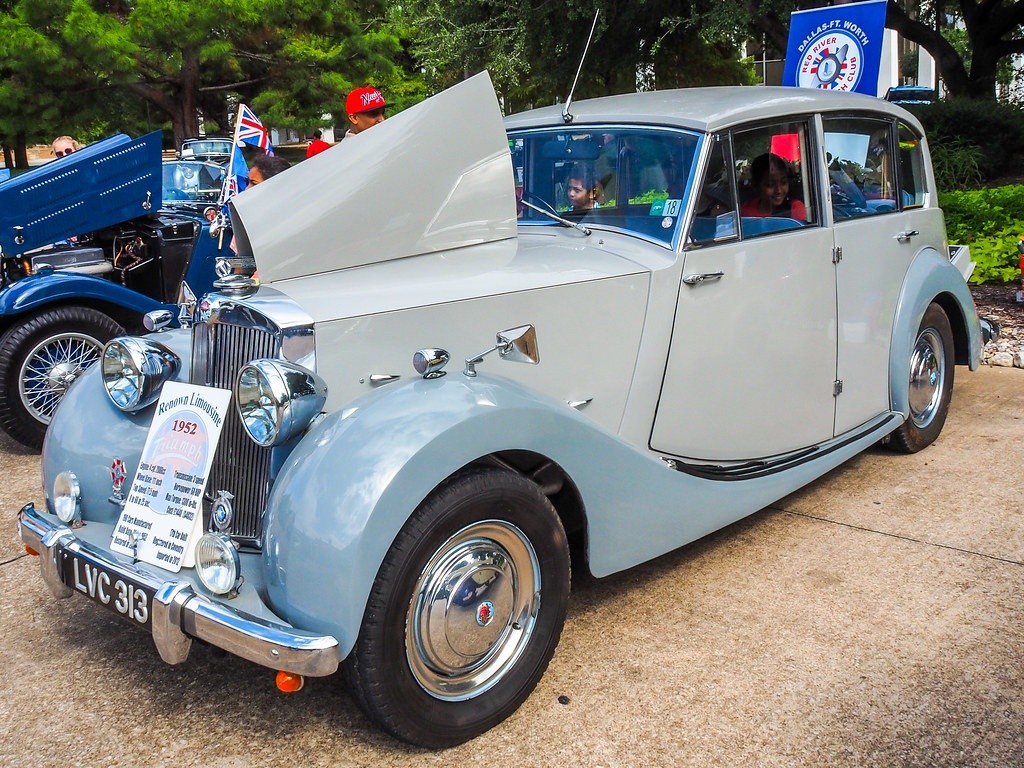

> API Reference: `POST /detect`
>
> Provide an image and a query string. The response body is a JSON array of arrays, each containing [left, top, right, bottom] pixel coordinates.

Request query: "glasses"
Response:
[[56, 148, 76, 158]]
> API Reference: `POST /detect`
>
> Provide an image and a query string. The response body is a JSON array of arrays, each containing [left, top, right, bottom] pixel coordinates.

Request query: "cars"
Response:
[[171, 139, 235, 201], [0, 128, 251, 451], [22, 68, 1005, 748]]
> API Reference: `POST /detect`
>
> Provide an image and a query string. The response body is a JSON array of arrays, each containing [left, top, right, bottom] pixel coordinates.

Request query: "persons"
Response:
[[663, 139, 741, 217], [567, 170, 600, 211], [341, 88, 395, 143], [304, 131, 331, 159], [50, 135, 80, 158], [830, 171, 856, 192], [229, 157, 294, 274], [739, 154, 806, 224]]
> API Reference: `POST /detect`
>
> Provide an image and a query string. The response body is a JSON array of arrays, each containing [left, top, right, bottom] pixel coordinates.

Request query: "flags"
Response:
[[222, 175, 238, 223], [239, 106, 274, 157], [231, 145, 250, 190]]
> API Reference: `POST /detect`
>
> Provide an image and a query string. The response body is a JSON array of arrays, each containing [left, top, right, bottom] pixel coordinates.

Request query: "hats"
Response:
[[345, 87, 396, 116]]
